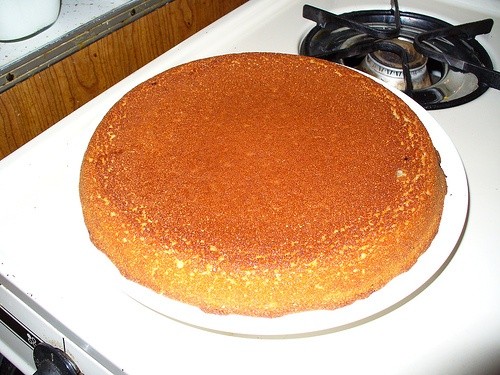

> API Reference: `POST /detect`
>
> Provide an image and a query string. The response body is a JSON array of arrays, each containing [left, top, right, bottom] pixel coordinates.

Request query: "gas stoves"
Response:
[[0, 0, 500, 375]]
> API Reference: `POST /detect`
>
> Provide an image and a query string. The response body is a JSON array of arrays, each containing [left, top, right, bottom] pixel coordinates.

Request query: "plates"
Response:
[[70, 61, 470, 336]]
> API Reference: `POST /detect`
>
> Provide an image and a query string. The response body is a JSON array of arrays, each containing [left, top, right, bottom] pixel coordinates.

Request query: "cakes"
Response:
[[75, 49, 446, 318]]
